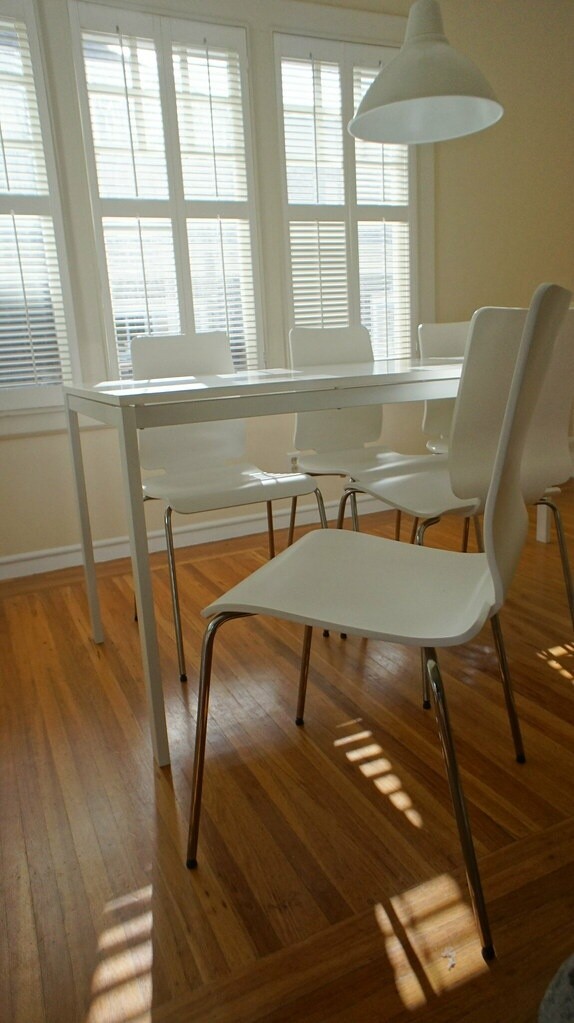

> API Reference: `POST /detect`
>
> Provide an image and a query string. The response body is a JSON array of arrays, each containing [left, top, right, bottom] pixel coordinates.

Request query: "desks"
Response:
[[61, 356, 552, 768]]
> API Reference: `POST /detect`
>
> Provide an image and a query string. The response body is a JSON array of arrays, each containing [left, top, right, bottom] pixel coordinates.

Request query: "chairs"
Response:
[[416, 325, 474, 549], [288, 328, 418, 544], [323, 303, 573, 712], [187, 283, 572, 964], [130, 332, 331, 680]]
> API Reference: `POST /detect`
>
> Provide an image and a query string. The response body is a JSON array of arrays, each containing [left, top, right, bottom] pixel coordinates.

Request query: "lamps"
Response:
[[348, 0, 505, 145]]
